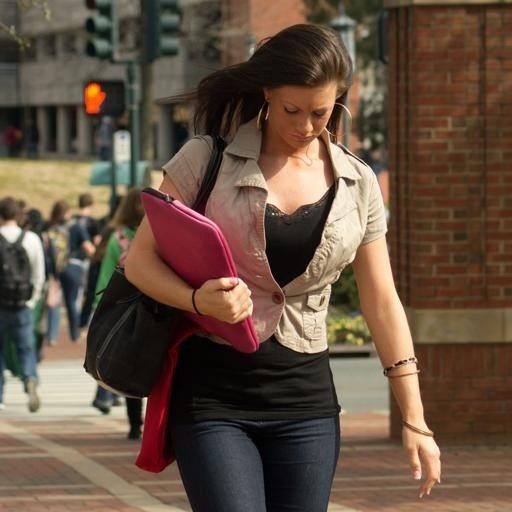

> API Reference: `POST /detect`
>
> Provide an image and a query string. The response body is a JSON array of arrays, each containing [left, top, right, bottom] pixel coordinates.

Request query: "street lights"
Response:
[[330, 0, 360, 154]]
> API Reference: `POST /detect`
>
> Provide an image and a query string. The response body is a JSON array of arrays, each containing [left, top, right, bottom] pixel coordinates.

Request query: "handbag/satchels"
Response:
[[140, 186, 259, 353], [45, 276, 65, 309], [84, 263, 191, 399], [137, 324, 199, 476]]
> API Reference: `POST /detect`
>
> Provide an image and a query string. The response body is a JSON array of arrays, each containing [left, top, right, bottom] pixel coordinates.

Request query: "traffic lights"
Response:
[[140, 1, 182, 65], [85, 0, 119, 64], [84, 79, 125, 117]]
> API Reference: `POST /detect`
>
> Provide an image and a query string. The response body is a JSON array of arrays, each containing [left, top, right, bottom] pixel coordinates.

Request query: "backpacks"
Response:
[[1, 230, 34, 310]]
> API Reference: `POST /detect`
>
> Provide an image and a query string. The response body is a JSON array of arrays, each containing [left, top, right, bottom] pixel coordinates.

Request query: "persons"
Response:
[[123, 21, 444, 512], [1, 189, 149, 444]]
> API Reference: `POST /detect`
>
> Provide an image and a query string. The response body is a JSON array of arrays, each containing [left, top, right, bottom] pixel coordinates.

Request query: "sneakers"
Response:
[[129, 426, 139, 438], [26, 377, 40, 412], [91, 400, 110, 414]]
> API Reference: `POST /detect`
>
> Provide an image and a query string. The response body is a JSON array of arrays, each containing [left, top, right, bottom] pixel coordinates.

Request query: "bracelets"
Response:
[[381, 355, 421, 376], [387, 369, 421, 381], [191, 286, 203, 320], [399, 416, 436, 438]]
